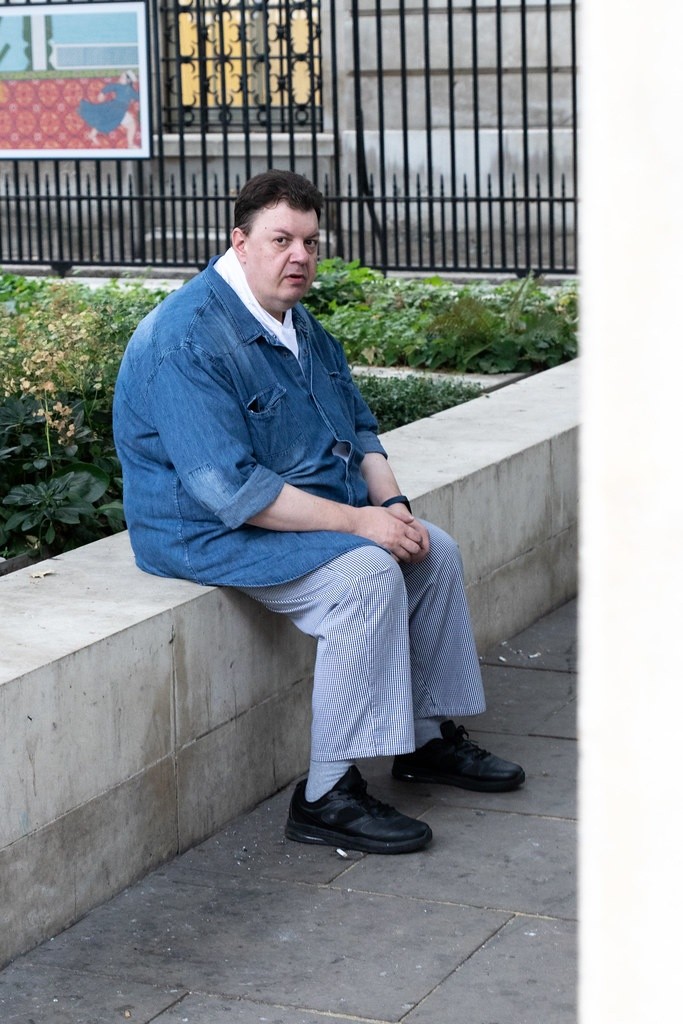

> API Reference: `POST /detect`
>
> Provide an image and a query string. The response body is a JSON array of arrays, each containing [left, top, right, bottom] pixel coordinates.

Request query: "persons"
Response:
[[113, 168, 526, 851]]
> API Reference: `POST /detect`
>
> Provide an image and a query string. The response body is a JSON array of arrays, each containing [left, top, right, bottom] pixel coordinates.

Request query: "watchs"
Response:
[[382, 496, 412, 515]]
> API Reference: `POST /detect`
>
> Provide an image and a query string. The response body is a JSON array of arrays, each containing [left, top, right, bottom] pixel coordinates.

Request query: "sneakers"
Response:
[[392, 720, 525, 791], [285, 765, 433, 854]]
